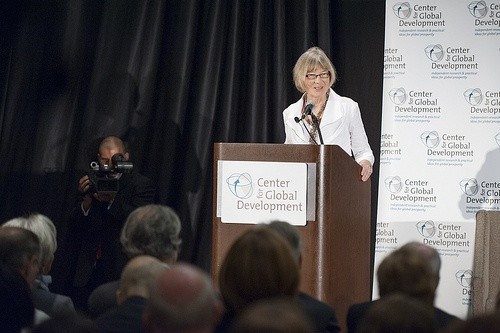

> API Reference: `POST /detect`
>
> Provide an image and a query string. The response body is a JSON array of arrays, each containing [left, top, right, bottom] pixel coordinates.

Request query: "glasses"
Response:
[[306, 73, 328, 78]]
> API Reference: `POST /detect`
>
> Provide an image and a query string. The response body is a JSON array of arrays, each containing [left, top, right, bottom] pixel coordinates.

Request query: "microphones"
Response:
[[301, 99, 315, 119]]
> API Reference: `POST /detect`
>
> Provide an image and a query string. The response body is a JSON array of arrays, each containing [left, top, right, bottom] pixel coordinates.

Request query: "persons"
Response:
[[0, 204, 500, 333], [49, 136, 160, 309], [283, 47, 375, 182]]
